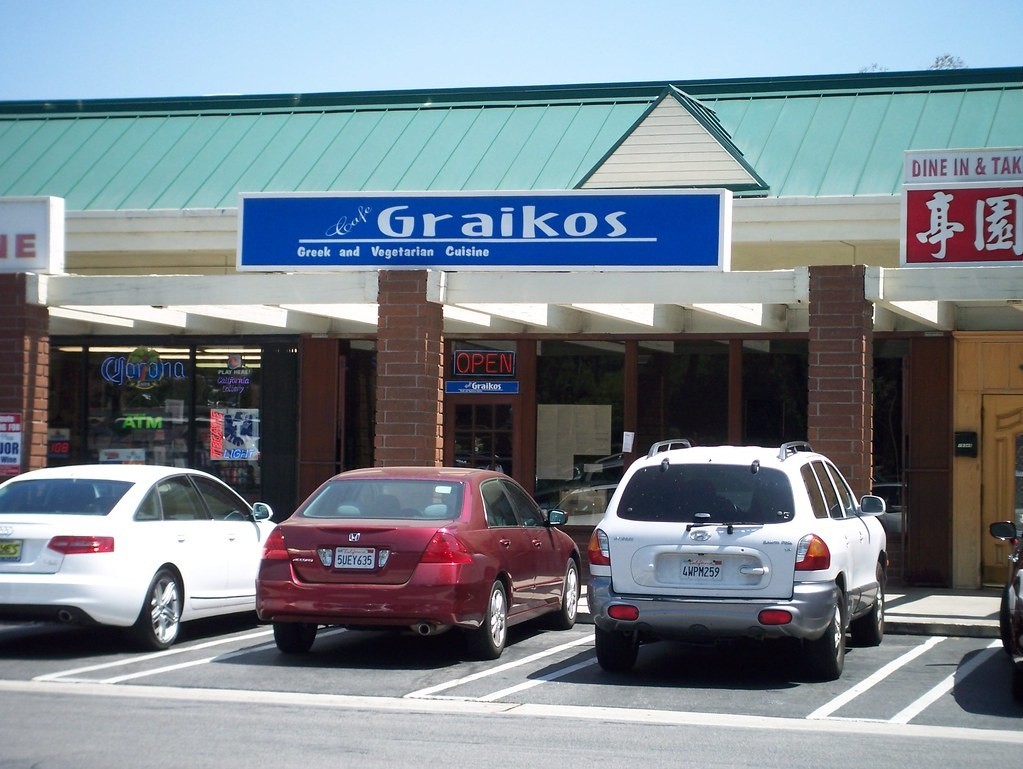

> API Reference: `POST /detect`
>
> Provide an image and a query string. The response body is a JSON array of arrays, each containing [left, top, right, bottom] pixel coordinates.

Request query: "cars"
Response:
[[535, 448, 623, 526], [989, 520, 1023, 701], [0, 463, 279, 652], [871, 476, 907, 536], [255, 466, 583, 661]]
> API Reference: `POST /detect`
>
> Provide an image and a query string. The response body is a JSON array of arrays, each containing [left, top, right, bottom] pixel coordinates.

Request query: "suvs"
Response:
[[586, 439, 889, 681]]
